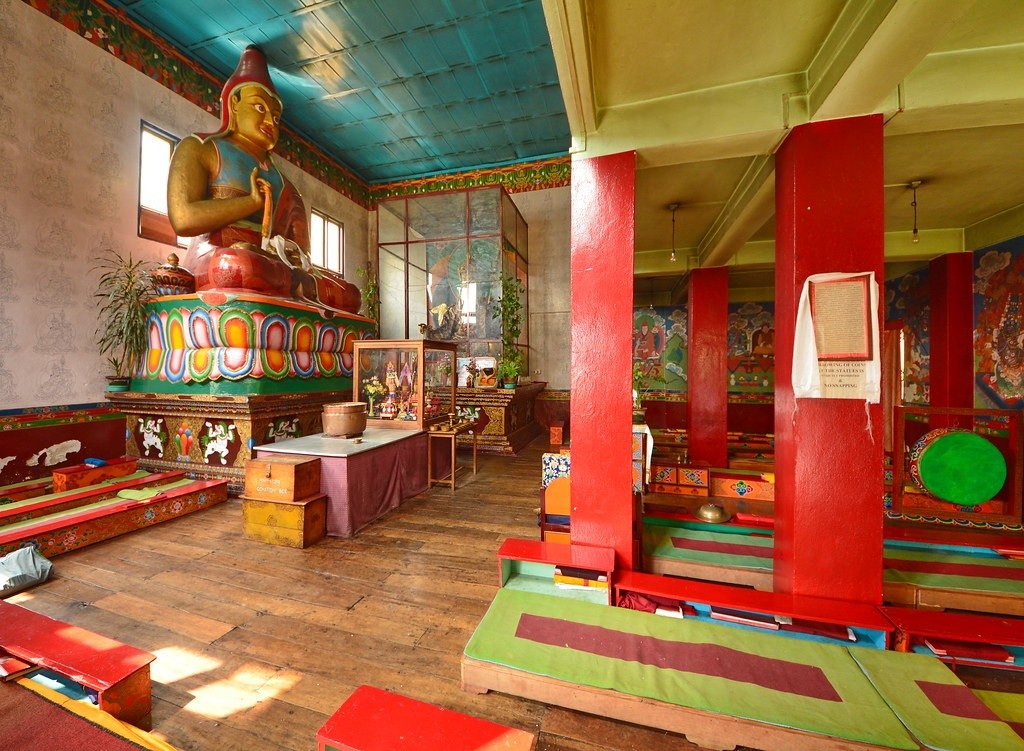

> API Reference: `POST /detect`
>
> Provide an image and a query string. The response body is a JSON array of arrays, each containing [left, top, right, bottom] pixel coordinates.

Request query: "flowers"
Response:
[[362, 379, 386, 397], [437, 356, 452, 372]]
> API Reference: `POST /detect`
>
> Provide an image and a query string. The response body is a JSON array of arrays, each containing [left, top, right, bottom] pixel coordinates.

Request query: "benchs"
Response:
[[550, 420, 565, 445]]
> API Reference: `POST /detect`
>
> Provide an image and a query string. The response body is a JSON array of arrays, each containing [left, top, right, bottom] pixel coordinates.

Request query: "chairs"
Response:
[[537, 453, 570, 542]]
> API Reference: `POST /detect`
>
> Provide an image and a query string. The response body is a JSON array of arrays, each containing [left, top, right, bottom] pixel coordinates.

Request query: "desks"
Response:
[[253, 428, 428, 538], [497, 538, 614, 605], [0, 597, 157, 727], [882, 607, 1024, 673], [616, 568, 895, 649], [428, 422, 480, 489], [315, 685, 539, 751]]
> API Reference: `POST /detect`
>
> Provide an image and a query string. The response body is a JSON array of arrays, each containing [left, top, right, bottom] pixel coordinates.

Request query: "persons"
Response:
[[165, 46, 363, 321]]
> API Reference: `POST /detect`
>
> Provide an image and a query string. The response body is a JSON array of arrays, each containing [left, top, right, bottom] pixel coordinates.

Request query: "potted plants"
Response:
[[632, 384, 650, 425], [492, 273, 525, 389], [88, 248, 163, 391]]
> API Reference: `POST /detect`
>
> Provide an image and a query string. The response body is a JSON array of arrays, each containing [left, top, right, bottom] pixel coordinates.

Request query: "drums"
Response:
[[908, 426, 1006, 505]]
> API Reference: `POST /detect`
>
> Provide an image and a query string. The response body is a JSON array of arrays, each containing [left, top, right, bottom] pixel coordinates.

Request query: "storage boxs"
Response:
[[246, 457, 322, 500], [241, 493, 329, 548]]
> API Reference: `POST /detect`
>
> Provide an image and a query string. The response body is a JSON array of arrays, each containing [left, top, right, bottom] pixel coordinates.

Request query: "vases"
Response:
[[442, 371, 448, 388], [369, 397, 375, 416]]
